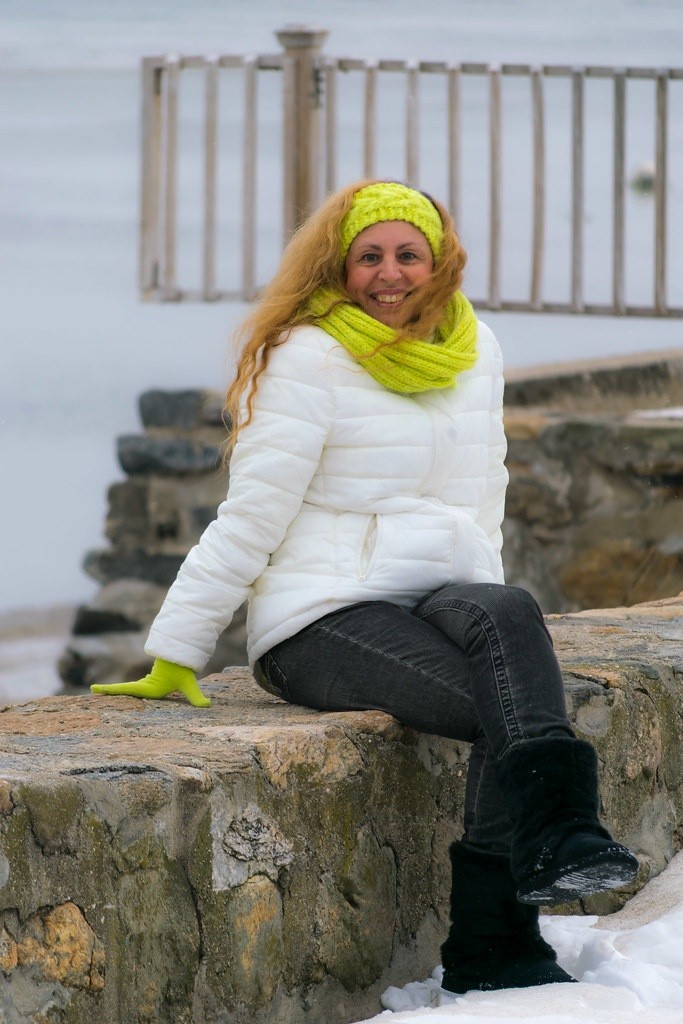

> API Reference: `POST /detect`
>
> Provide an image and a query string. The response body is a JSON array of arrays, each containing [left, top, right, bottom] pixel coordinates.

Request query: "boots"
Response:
[[502, 736, 639, 905], [442, 840, 579, 995]]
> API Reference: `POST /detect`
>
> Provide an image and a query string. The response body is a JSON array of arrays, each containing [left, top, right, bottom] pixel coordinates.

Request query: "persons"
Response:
[[87, 177, 642, 994]]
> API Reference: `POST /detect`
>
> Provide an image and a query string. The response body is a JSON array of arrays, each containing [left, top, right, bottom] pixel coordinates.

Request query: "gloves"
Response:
[[90, 657, 211, 707]]
